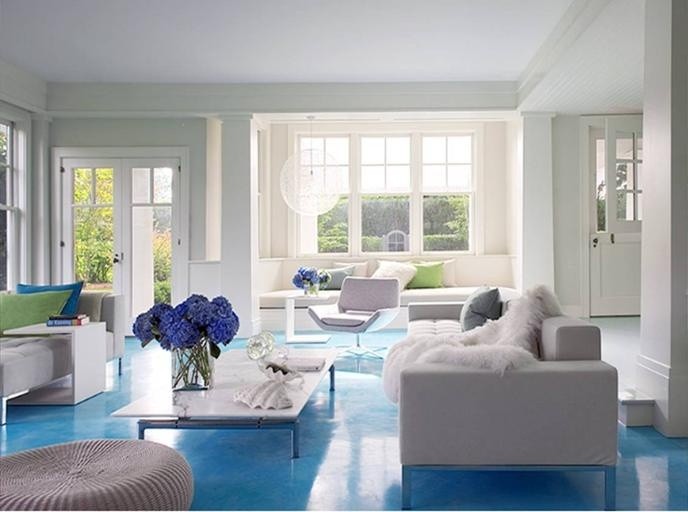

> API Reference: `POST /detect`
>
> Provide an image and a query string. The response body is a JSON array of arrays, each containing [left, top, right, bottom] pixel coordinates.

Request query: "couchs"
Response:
[[0, 285, 126, 426], [383, 299, 619, 509], [261, 255, 511, 331]]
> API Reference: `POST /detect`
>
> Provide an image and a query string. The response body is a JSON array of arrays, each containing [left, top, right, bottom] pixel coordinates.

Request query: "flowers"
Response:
[[132, 292, 240, 386], [290, 265, 332, 293]]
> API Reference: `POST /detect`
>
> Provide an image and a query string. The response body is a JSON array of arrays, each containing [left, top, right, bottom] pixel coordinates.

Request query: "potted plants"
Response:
[[76, 218, 117, 289]]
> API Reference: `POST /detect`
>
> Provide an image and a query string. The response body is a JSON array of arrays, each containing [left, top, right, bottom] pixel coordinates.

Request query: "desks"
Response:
[[4, 320, 107, 408], [283, 294, 333, 346]]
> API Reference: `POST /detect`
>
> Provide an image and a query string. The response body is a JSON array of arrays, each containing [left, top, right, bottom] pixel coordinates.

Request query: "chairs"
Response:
[[307, 277, 402, 361]]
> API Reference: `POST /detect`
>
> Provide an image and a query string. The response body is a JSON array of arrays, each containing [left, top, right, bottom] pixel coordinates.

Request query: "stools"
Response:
[[0, 439, 195, 510]]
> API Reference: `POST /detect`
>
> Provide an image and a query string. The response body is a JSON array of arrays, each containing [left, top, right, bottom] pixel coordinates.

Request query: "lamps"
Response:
[[278, 113, 343, 217]]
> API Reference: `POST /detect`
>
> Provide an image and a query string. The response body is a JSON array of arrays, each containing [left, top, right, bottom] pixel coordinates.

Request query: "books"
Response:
[[47, 314, 90, 327]]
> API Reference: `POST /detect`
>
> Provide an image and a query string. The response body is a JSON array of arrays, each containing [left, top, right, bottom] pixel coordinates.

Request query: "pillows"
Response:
[[458, 285, 501, 331], [319, 259, 456, 291], [1, 289, 74, 334], [16, 280, 84, 315]]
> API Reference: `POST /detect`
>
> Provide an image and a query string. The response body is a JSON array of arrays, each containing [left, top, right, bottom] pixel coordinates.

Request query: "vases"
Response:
[[303, 282, 320, 298], [168, 338, 216, 393]]
